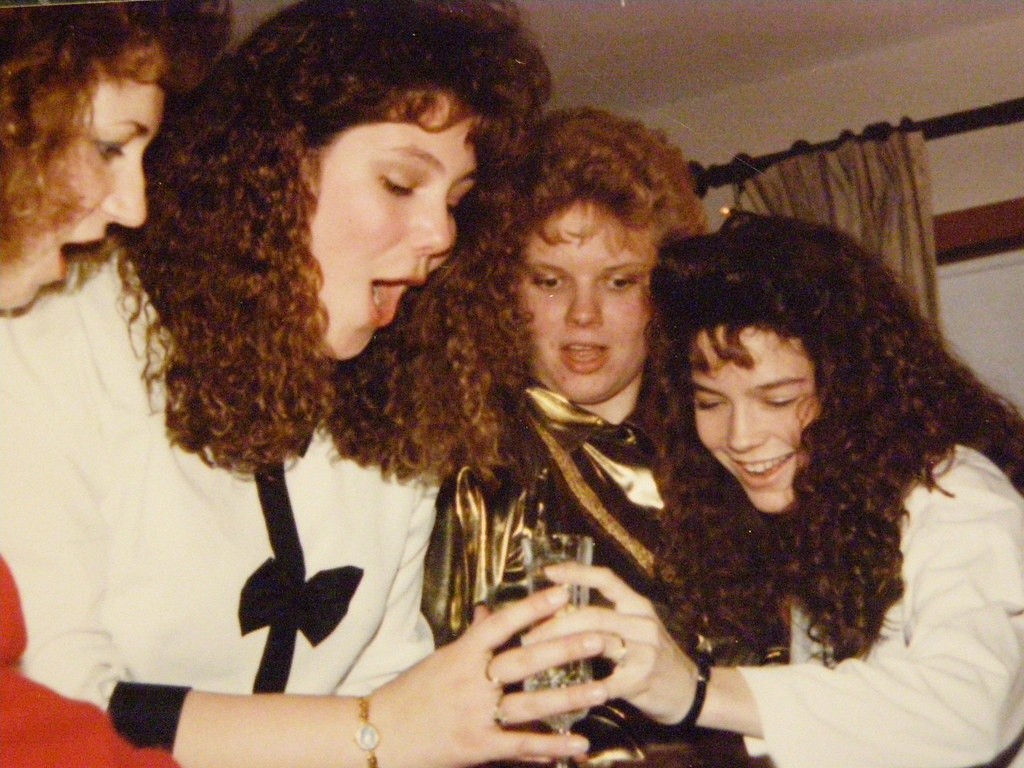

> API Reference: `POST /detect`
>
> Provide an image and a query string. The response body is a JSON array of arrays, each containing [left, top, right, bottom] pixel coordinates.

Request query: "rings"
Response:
[[485, 656, 506, 688], [613, 634, 628, 662], [497, 694, 508, 728]]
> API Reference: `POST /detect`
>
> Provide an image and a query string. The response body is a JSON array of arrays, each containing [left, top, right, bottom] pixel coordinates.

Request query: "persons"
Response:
[[0, 0, 555, 768], [419, 104, 758, 768], [522, 212, 1024, 768]]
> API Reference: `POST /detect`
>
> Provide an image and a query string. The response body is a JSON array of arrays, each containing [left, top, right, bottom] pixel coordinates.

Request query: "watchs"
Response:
[[357, 695, 379, 768]]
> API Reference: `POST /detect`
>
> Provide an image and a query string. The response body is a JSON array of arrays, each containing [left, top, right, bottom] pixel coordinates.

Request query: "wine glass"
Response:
[[523, 534, 594, 768]]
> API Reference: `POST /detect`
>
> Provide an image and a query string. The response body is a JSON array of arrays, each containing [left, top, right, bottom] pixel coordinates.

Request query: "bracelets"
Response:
[[662, 659, 711, 732]]
